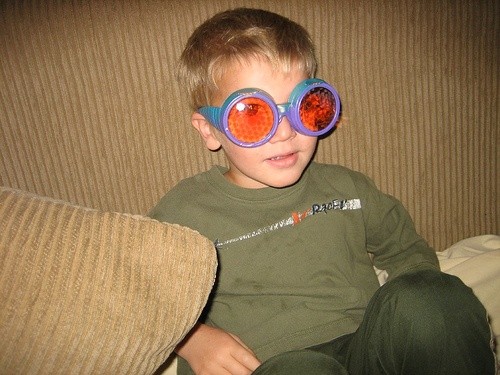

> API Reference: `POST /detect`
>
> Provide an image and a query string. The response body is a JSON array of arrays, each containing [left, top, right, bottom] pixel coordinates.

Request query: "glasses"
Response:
[[197, 78, 340, 148]]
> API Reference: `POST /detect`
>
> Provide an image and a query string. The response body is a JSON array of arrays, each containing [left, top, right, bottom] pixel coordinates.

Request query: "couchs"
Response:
[[0, 1, 500, 375]]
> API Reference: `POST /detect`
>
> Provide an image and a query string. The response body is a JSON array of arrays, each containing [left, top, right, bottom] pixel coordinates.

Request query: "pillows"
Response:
[[3, 184, 220, 374]]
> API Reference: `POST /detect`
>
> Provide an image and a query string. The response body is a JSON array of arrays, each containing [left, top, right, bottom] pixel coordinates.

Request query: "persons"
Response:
[[146, 8, 495, 375]]
